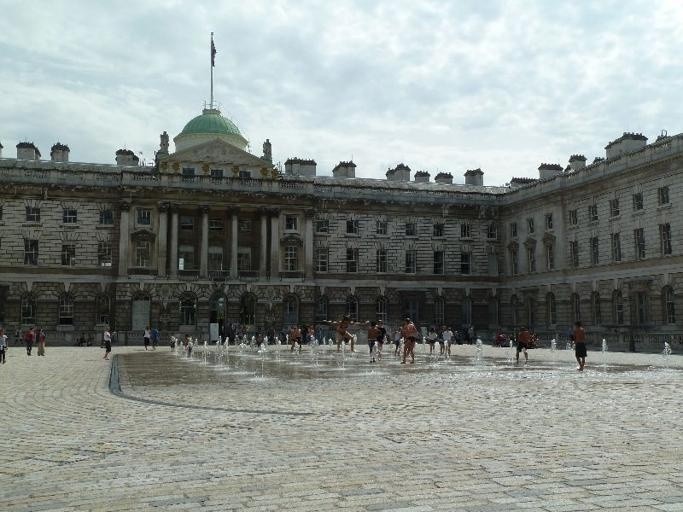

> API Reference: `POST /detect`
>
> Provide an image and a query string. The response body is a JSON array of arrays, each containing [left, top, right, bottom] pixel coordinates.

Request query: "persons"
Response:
[[491, 326, 539, 366], [0, 327, 46, 364], [334, 315, 475, 365], [566, 320, 587, 371], [103, 325, 115, 360], [228, 324, 315, 353], [143, 326, 193, 351]]
